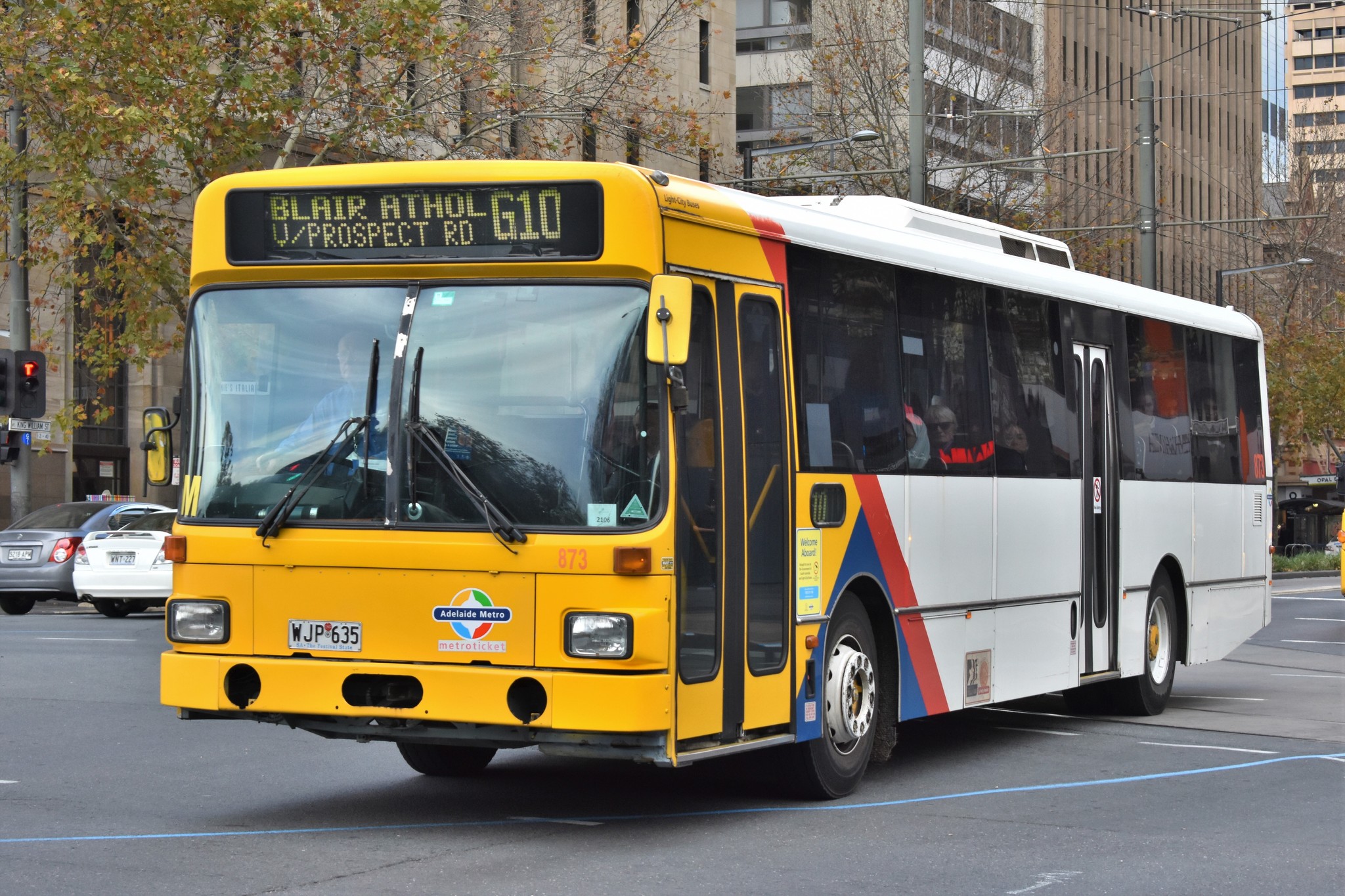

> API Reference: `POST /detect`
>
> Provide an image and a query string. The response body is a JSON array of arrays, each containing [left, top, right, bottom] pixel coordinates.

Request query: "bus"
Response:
[[140, 161, 1275, 799]]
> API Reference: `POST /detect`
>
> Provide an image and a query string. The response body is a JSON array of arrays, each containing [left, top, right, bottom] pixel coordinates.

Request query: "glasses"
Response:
[[925, 421, 955, 431]]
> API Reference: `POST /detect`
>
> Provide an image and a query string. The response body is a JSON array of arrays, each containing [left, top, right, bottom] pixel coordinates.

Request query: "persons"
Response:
[[828, 348, 1245, 483], [1277, 522, 1288, 546], [252, 326, 413, 491]]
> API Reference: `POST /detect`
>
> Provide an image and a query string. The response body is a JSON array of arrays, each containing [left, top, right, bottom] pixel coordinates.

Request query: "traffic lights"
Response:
[[0, 348, 52, 466]]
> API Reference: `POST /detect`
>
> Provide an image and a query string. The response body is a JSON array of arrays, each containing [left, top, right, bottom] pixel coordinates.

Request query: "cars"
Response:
[[0, 498, 171, 615], [72, 509, 181, 618]]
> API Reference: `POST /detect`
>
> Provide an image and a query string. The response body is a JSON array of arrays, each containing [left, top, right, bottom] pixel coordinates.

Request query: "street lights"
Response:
[[743, 130, 882, 193], [1216, 256, 1315, 307]]
